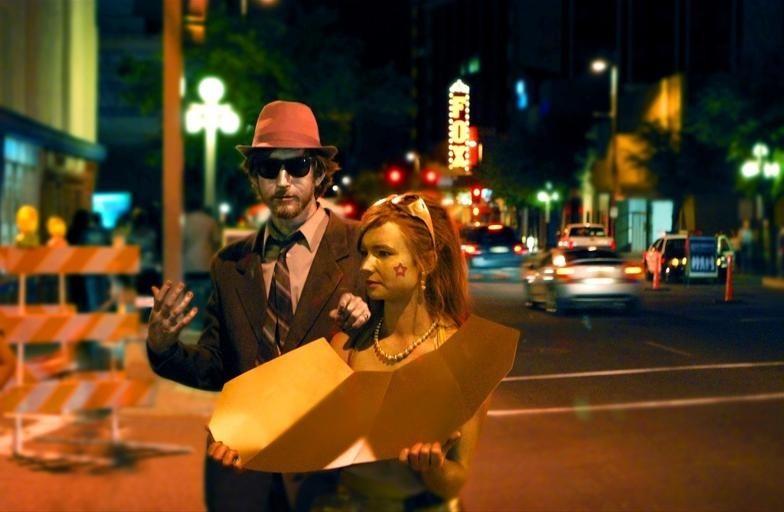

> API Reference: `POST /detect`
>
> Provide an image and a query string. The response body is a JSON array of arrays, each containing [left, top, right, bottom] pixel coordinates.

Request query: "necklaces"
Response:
[[373, 315, 442, 360]]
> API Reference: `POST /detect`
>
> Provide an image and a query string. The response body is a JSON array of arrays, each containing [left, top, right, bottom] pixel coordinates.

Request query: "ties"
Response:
[[263, 234, 301, 350]]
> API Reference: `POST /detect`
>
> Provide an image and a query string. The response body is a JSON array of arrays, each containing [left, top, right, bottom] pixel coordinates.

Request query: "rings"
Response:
[[363, 314, 369, 321]]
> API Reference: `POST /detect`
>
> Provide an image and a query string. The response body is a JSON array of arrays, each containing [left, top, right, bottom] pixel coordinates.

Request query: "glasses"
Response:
[[254, 151, 312, 179], [369, 193, 436, 252]]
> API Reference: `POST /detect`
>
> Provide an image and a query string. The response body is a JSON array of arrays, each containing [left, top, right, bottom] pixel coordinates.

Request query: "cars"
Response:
[[556, 223, 616, 251], [519, 243, 648, 315]]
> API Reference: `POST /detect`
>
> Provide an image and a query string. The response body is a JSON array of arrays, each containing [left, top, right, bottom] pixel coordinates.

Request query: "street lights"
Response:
[[739, 141, 782, 220], [332, 184, 342, 197], [536, 180, 559, 245], [589, 58, 623, 239], [182, 75, 242, 213], [406, 150, 421, 173]]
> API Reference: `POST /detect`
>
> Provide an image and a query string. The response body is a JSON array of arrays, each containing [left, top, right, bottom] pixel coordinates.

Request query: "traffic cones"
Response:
[[646, 255, 665, 292], [722, 254, 737, 305]]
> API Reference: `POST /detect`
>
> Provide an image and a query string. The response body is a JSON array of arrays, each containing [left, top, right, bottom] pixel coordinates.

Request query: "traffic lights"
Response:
[[471, 185, 481, 221]]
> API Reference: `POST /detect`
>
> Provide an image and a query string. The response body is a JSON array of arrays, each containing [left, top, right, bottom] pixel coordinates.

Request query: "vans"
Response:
[[454, 221, 529, 273]]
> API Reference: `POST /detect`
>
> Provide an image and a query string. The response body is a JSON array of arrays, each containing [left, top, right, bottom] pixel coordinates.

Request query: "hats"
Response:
[[234, 100, 338, 160]]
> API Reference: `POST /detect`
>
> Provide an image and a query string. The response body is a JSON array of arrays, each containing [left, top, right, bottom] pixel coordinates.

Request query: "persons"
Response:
[[146, 101, 380, 511], [729, 215, 772, 274], [62, 194, 223, 340], [206, 191, 491, 511]]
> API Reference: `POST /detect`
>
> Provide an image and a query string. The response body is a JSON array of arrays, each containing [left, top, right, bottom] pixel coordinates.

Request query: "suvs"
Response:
[[636, 229, 733, 288]]
